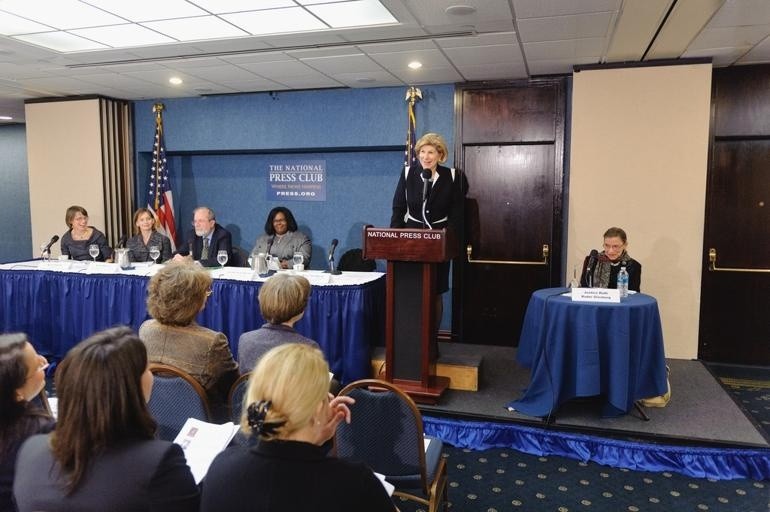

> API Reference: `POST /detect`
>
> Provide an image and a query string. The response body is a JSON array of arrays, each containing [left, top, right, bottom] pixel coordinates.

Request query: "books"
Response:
[[169, 413, 242, 490], [371, 468, 396, 499]]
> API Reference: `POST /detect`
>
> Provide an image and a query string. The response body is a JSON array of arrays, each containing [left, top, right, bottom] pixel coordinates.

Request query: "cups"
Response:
[[59, 255, 68, 266], [254, 253, 269, 272], [291, 251, 304, 273], [114, 248, 130, 270]]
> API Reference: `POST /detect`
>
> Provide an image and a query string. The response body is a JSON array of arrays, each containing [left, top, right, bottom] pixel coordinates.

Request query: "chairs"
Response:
[[142, 358, 216, 442], [226, 369, 252, 424], [330, 378, 451, 512]]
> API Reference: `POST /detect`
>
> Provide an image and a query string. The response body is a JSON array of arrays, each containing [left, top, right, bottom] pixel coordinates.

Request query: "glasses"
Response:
[[24, 353, 51, 384], [603, 243, 625, 250]]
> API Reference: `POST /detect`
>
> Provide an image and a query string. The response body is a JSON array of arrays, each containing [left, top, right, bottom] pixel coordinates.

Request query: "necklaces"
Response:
[[71, 231, 89, 239]]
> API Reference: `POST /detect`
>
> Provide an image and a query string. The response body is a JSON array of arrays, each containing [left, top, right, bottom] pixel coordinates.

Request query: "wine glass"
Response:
[[89, 245, 101, 265], [148, 247, 160, 266], [217, 250, 229, 270]]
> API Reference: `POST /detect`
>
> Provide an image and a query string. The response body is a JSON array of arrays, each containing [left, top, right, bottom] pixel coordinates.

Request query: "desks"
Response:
[[504, 285, 668, 429], [0, 256, 387, 391]]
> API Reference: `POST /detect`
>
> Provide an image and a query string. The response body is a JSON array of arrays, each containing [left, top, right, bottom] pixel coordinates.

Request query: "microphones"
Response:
[[177, 239, 195, 253], [42, 235, 59, 253], [109, 235, 127, 254], [422, 168, 432, 201], [586, 249, 597, 272], [266, 239, 274, 253], [328, 239, 338, 261]]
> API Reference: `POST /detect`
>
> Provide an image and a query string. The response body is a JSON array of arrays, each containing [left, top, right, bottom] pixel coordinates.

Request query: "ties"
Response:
[[200, 237, 210, 260]]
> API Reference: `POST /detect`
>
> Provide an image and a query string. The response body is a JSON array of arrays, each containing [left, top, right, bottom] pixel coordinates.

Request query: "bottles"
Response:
[[617, 267, 629, 297]]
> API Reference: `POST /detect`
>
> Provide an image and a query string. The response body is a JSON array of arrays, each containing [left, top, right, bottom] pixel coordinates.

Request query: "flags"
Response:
[[141, 103, 179, 259], [402, 86, 421, 166]]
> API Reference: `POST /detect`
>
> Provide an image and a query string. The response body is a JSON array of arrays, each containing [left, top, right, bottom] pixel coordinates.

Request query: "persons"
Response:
[[244, 205, 314, 277], [170, 207, 238, 269], [580, 225, 644, 299], [12, 325, 203, 511], [0, 328, 57, 511], [387, 132, 467, 364], [136, 259, 240, 414], [230, 272, 332, 383], [59, 204, 114, 264], [121, 207, 175, 263], [197, 341, 400, 510]]
